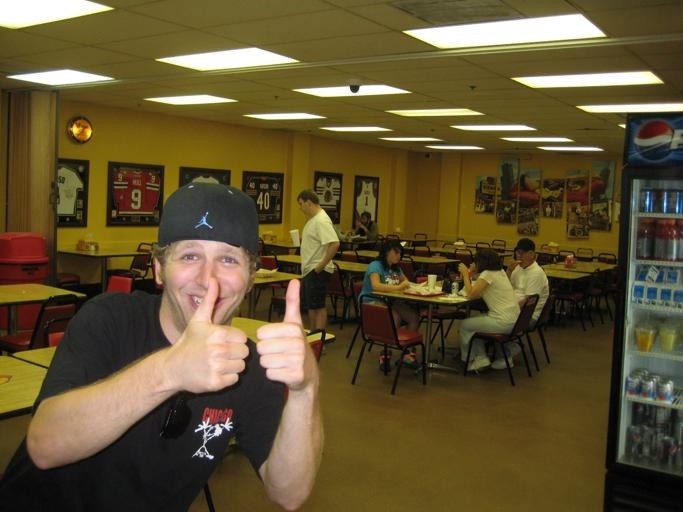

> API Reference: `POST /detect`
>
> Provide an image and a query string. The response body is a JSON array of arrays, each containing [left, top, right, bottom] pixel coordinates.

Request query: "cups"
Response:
[[289, 229, 300, 249], [428, 274, 437, 289]]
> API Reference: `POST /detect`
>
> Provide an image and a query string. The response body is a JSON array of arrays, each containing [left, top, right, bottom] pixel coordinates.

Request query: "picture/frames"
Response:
[[106, 161, 165, 227], [179, 166, 232, 190], [55, 158, 90, 227], [352, 175, 380, 229], [242, 170, 284, 225], [313, 170, 343, 225]]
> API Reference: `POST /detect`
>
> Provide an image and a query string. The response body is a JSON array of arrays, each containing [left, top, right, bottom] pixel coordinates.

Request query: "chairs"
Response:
[[1, 239, 164, 349]]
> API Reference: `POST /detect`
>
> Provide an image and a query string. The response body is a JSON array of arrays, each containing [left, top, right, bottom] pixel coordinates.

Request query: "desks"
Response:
[[0, 283, 88, 356], [0, 356, 48, 421], [12, 315, 335, 512]]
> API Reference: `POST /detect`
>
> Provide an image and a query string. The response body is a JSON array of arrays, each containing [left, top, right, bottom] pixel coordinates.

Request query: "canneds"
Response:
[[626, 368, 674, 402]]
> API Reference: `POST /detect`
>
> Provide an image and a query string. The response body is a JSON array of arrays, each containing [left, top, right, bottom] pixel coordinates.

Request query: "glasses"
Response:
[[159, 393, 194, 440]]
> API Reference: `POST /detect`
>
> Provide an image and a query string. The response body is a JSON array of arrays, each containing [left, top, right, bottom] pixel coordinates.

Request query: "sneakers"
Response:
[[491, 356, 514, 369], [467, 352, 490, 372], [400, 347, 420, 368], [379, 350, 392, 372]]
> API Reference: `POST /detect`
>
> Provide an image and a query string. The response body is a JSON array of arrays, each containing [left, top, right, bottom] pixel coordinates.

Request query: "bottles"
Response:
[[604, 112, 683, 510], [634, 315, 682, 352], [637, 219, 682, 261], [451, 279, 459, 298]]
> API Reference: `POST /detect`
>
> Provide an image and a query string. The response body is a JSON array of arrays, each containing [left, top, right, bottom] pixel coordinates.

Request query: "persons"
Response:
[[569, 223, 576, 236], [457, 246, 525, 371], [296, 189, 341, 356], [520, 167, 611, 214], [361, 239, 422, 372], [546, 204, 552, 217], [0, 181, 326, 512], [475, 198, 486, 212], [353, 211, 380, 241], [328, 210, 350, 243], [490, 238, 550, 370]]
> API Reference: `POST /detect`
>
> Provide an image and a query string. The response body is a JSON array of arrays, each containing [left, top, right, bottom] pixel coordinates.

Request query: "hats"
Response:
[[158, 182, 258, 254], [514, 238, 535, 251]]
